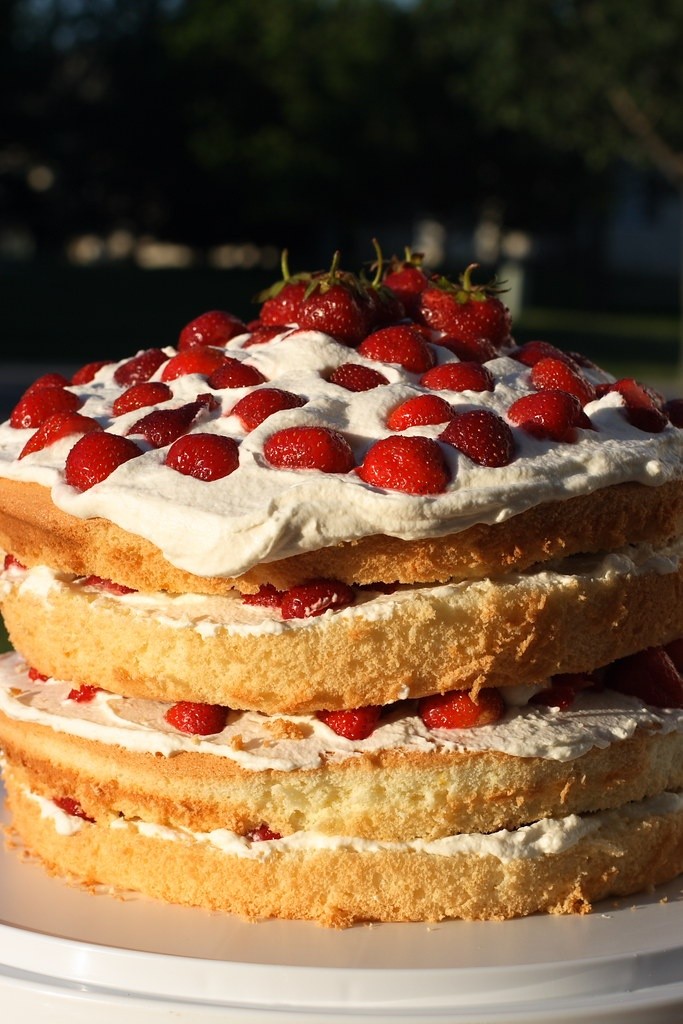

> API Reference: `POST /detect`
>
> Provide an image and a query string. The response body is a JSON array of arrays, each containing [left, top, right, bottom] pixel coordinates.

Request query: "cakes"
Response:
[[1, 241, 682, 923]]
